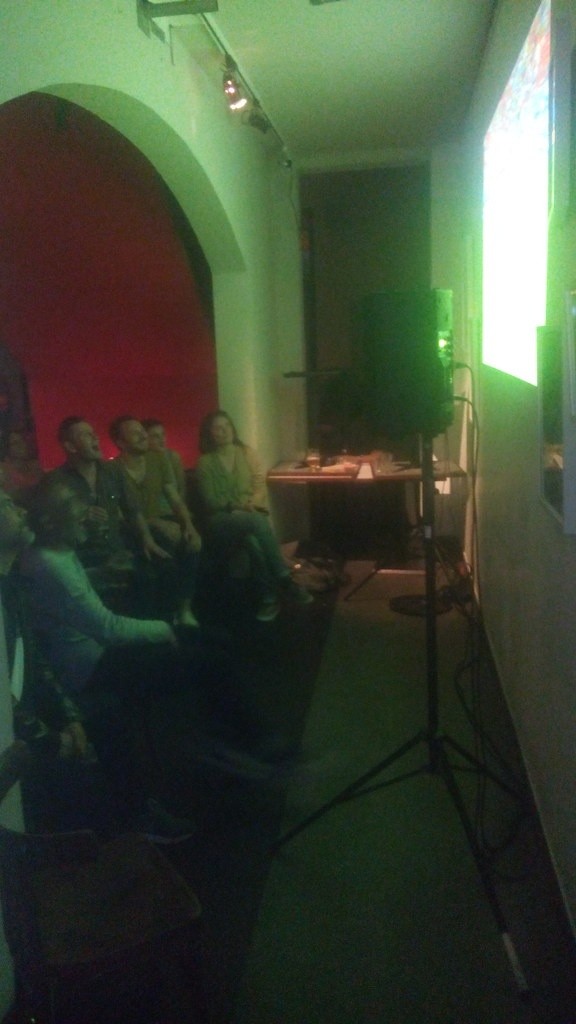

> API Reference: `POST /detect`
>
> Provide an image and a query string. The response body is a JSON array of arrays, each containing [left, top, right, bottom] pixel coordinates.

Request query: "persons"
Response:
[[0, 415, 202, 805], [191, 410, 315, 623]]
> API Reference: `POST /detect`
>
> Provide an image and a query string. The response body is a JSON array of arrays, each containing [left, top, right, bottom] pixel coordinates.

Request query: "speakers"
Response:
[[361, 288, 456, 435]]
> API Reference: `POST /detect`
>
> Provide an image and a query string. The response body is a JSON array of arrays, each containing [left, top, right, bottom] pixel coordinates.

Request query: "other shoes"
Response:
[[286, 581, 314, 605], [255, 600, 281, 622], [177, 607, 200, 633]]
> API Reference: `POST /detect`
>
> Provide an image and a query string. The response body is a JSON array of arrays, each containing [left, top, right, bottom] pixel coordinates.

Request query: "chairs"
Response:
[[0, 826, 212, 1023]]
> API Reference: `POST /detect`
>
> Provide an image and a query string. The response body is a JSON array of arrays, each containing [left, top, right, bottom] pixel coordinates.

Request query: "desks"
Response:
[[267, 456, 467, 560]]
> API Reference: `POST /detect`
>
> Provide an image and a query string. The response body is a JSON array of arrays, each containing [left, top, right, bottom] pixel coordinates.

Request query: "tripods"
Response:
[[274, 434, 534, 990]]
